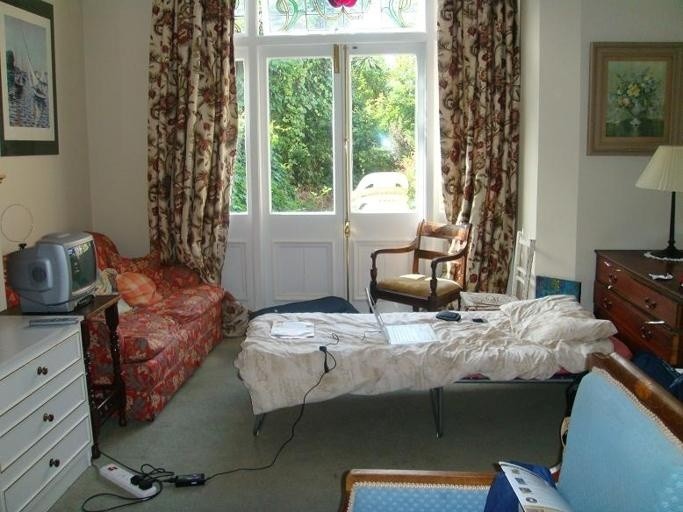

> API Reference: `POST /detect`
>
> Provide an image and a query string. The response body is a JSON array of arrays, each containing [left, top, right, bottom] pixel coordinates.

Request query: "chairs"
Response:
[[340, 351, 683, 511], [349, 169, 410, 214], [458, 228, 536, 311], [369, 219, 472, 313]]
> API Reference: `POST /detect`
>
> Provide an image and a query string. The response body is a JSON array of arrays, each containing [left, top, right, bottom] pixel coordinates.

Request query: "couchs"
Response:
[[4, 231, 224, 422]]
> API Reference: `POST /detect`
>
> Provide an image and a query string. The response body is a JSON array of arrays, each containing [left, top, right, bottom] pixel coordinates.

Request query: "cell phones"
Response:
[[436, 310, 460, 321]]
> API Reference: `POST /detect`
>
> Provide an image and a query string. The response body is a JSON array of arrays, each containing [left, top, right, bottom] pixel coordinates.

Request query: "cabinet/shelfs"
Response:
[[594, 249, 683, 366], [1, 315, 95, 512]]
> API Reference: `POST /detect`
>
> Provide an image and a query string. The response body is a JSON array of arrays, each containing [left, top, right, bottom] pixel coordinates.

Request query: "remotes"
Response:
[[29, 318, 78, 326]]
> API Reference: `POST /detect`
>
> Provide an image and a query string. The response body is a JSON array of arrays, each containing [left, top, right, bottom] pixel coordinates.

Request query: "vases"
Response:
[[629, 100, 643, 127]]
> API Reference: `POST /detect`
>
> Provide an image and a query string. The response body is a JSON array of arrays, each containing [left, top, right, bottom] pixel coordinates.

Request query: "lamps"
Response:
[[633, 145, 683, 261]]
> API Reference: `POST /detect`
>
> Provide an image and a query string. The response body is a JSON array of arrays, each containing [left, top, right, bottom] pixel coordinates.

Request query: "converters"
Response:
[[176, 473, 205, 487]]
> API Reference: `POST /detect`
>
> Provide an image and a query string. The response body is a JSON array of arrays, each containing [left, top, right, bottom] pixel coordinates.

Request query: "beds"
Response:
[[237, 292, 633, 437]]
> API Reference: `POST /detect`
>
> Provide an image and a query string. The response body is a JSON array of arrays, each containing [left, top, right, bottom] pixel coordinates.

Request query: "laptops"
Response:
[[366, 288, 440, 344]]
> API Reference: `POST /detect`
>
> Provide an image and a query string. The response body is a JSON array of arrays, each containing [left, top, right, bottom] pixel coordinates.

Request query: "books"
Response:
[[270, 318, 317, 340], [498, 459, 573, 512]]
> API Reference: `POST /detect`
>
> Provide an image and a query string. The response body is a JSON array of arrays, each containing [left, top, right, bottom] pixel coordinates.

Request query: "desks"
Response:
[[1, 295, 129, 458]]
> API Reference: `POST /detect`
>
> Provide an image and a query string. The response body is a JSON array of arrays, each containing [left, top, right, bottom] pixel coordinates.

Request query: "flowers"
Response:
[[609, 66, 662, 116]]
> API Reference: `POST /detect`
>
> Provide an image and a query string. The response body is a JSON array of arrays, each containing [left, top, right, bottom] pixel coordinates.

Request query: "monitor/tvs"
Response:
[[8, 230, 98, 312]]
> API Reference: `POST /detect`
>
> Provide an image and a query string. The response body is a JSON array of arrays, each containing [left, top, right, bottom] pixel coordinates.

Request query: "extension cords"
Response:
[[99, 464, 157, 500]]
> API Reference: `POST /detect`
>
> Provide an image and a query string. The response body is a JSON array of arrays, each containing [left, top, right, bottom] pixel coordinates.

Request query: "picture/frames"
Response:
[[0, 0, 59, 156], [587, 42, 683, 157]]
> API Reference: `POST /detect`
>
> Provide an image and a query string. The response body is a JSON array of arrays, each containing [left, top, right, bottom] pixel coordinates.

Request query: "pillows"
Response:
[[499, 294, 619, 347], [117, 271, 162, 308]]
